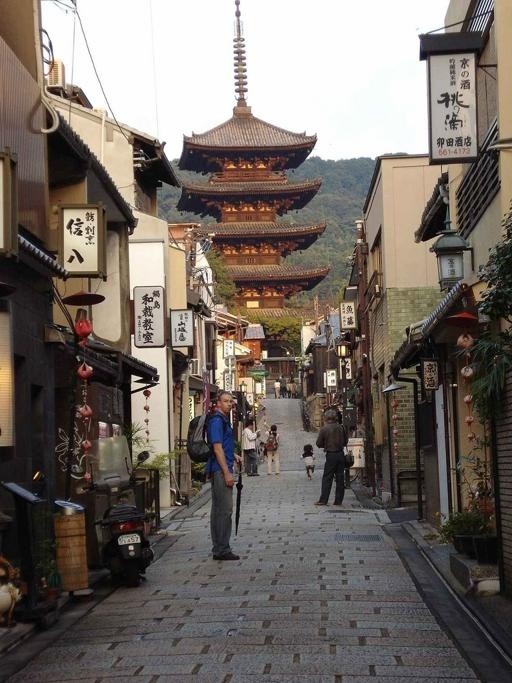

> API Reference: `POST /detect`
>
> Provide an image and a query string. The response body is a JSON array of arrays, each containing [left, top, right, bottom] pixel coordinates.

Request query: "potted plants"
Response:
[[44, 572, 60, 602], [423, 499, 500, 567]]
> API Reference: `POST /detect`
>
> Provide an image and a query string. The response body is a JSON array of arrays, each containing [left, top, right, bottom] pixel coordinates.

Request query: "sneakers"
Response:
[[212, 551, 239, 559]]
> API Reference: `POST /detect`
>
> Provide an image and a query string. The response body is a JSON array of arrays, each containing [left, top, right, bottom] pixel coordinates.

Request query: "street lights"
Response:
[[336, 339, 352, 487], [240, 381, 248, 429]]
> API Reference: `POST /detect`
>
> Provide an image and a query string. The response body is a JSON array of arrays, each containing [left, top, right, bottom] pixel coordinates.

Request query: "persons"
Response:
[[273, 376, 298, 398], [264, 424, 282, 476], [241, 420, 261, 478], [300, 442, 317, 480], [313, 408, 349, 506], [203, 389, 245, 560]]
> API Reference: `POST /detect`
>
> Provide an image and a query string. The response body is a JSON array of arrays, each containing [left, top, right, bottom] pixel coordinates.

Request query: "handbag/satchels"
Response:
[[345, 455, 355, 468]]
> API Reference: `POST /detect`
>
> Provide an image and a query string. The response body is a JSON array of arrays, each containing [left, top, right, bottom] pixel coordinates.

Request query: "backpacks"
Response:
[[187, 414, 225, 461], [265, 431, 278, 450]]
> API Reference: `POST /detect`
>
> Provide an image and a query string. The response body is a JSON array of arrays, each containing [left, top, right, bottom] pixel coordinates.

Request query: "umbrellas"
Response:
[[232, 462, 243, 535]]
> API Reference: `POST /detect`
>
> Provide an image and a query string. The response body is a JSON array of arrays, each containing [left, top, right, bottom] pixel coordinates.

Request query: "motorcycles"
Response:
[[81, 451, 154, 588]]
